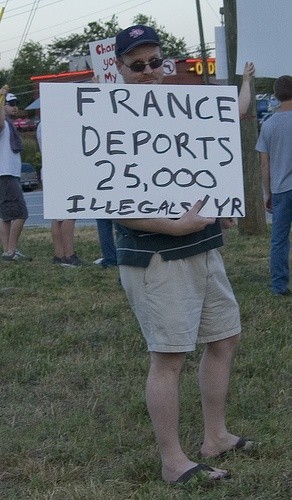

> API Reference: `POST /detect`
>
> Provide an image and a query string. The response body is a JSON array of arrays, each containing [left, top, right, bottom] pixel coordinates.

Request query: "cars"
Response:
[[12, 109, 40, 132], [256, 92, 280, 131]]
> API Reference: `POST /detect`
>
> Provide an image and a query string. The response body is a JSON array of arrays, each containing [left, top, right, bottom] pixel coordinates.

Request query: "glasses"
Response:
[[5, 102, 19, 107], [118, 55, 165, 72]]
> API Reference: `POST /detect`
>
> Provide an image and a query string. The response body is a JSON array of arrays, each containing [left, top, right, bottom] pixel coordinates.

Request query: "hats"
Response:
[[6, 92, 17, 101], [115, 25, 161, 56]]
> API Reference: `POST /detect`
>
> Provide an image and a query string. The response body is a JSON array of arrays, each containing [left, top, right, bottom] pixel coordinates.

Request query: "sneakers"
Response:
[[53, 250, 90, 268], [0, 249, 33, 263]]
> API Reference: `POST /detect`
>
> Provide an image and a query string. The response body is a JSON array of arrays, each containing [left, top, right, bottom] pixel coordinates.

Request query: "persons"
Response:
[[0, 85, 29, 262], [237, 61, 255, 120], [113, 26, 261, 483], [36, 122, 117, 266], [255, 75, 292, 295]]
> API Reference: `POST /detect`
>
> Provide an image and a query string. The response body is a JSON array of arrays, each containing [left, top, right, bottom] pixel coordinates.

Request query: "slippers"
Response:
[[196, 436, 262, 461], [162, 463, 235, 488]]
